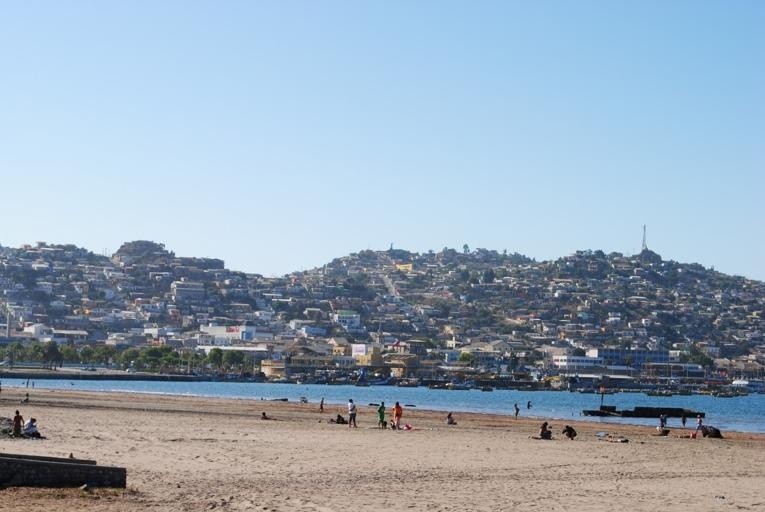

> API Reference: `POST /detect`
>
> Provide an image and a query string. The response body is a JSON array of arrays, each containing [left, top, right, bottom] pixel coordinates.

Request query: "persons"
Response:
[[19, 393, 29, 404], [538, 421, 551, 439], [319, 396, 403, 430], [514, 403, 520, 420], [527, 400, 533, 409], [261, 412, 284, 421], [660, 413, 702, 434], [561, 425, 577, 440], [12, 410, 24, 436], [447, 412, 457, 425], [23, 417, 40, 436]]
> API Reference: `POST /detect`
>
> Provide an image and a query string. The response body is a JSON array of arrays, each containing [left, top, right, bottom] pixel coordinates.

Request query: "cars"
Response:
[[81, 366, 97, 372]]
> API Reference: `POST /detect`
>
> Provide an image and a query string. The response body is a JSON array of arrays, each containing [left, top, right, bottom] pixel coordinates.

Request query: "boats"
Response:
[[582, 406, 706, 418]]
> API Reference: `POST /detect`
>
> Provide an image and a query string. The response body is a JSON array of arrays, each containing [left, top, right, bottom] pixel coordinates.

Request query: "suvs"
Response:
[[126, 368, 136, 372]]
